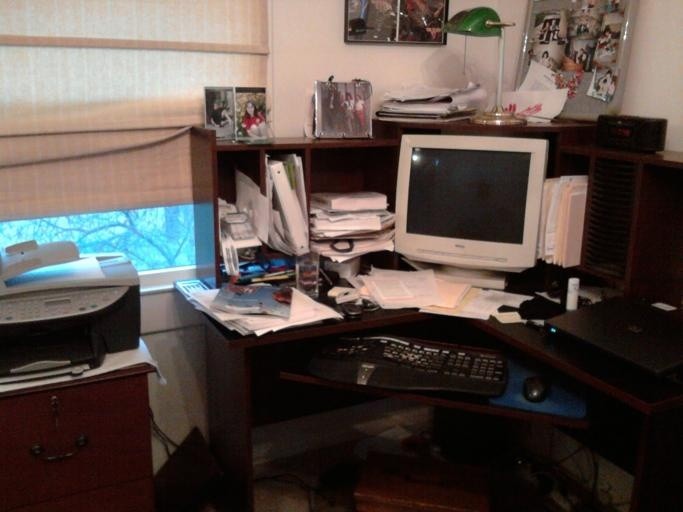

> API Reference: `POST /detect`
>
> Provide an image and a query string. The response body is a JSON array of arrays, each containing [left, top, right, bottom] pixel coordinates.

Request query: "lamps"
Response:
[[445, 6, 528, 126]]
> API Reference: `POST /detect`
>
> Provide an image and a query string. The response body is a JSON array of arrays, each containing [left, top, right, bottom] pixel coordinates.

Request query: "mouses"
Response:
[[524, 376, 551, 402]]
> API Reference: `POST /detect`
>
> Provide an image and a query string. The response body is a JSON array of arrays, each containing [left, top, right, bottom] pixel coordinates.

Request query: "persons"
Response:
[[240, 101, 266, 136], [528, 6, 624, 101], [323, 84, 368, 136], [208, 95, 233, 133]]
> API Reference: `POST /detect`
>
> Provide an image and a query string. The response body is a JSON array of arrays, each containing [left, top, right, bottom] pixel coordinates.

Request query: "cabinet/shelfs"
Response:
[[0, 337, 157, 512]]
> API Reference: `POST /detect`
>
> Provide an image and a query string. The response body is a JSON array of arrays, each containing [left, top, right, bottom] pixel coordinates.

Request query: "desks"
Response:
[[174, 117, 682, 512]]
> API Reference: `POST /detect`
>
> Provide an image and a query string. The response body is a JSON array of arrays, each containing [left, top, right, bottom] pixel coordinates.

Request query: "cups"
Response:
[[295, 242, 320, 299]]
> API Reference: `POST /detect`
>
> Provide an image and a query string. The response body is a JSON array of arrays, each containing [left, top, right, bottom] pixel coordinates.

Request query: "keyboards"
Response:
[[306, 335, 508, 397]]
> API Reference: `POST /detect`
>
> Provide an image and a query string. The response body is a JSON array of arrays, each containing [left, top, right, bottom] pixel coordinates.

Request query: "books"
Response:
[[185, 150, 398, 337], [373, 80, 488, 124], [536, 175, 589, 268]]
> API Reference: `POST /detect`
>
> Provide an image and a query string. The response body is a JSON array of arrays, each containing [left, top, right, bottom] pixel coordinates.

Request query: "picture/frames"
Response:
[[345, 0, 448, 46], [234, 86, 268, 142], [206, 86, 234, 140]]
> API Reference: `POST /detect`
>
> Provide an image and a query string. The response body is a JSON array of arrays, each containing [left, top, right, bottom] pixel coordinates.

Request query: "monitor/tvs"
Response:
[[394, 134, 549, 293]]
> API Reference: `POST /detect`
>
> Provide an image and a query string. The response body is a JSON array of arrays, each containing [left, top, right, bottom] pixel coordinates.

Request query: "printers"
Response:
[[0, 239, 141, 377]]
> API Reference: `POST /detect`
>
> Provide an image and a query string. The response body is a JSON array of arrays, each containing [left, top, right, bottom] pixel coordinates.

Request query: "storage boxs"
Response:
[[352, 451, 488, 511]]
